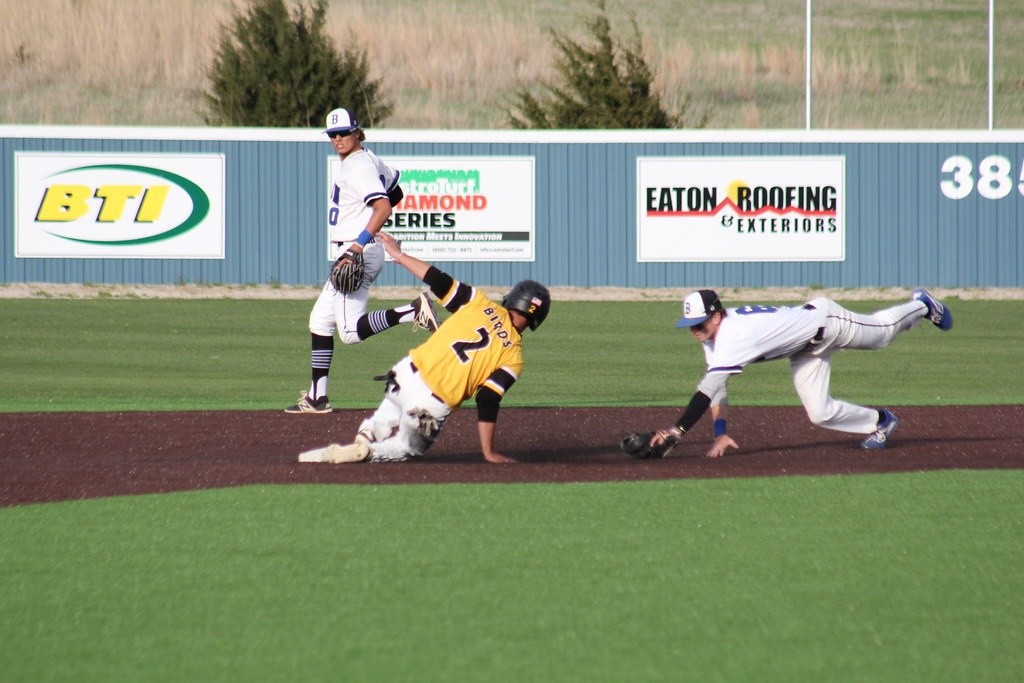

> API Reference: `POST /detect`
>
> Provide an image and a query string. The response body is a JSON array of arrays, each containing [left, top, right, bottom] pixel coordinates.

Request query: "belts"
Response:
[[337, 237, 374, 248], [801, 304, 823, 353]]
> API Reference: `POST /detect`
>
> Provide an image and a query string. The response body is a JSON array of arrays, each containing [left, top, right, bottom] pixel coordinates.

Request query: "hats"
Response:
[[321, 107, 358, 133], [675, 290, 721, 327]]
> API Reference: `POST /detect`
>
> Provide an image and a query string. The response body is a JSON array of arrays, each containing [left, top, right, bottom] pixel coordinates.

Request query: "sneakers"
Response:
[[912, 288, 952, 331], [861, 408, 899, 449], [284, 390, 333, 414], [411, 292, 442, 334]]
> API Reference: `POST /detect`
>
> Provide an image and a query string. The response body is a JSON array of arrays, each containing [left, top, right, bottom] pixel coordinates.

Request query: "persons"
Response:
[[298, 232, 550, 462], [649, 288, 952, 458], [284, 108, 440, 413]]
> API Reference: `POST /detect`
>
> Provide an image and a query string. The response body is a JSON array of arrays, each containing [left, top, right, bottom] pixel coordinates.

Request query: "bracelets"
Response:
[[396, 253, 405, 263]]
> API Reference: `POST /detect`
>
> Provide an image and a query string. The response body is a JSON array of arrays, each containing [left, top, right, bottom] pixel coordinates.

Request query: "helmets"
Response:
[[503, 280, 551, 331]]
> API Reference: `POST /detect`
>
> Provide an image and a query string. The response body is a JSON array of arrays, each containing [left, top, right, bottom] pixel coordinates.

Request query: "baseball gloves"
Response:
[[328, 251, 366, 294], [620, 432, 680, 457]]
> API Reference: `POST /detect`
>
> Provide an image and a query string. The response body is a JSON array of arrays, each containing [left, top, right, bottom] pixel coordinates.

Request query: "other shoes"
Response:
[[323, 444, 373, 464], [355, 429, 376, 444]]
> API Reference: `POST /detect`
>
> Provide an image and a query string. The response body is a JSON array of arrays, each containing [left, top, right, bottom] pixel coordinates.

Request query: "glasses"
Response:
[[327, 128, 356, 138], [704, 296, 721, 313]]
